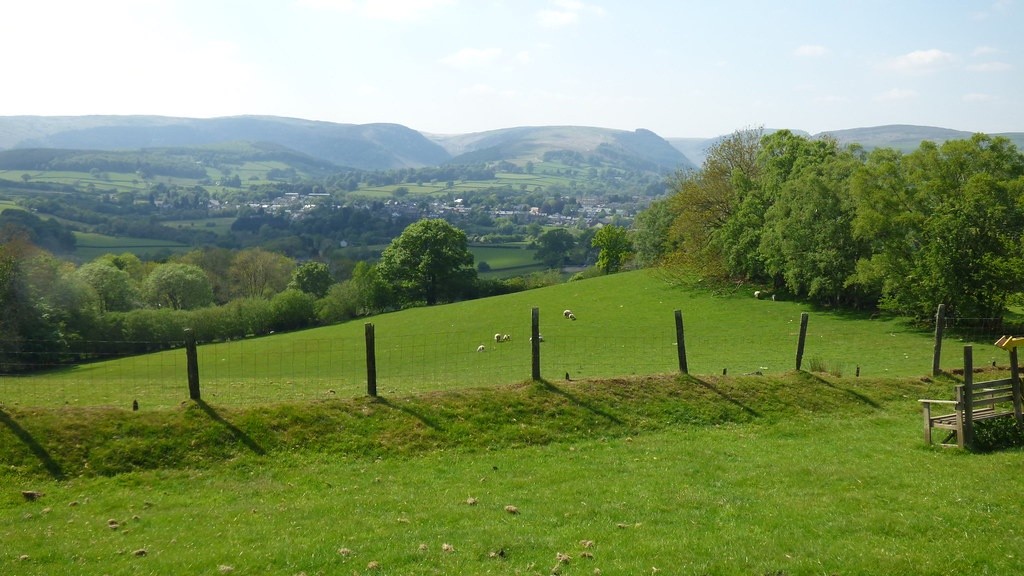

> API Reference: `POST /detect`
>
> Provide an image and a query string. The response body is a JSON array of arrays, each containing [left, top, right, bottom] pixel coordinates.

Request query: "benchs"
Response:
[[919, 377, 1024, 450]]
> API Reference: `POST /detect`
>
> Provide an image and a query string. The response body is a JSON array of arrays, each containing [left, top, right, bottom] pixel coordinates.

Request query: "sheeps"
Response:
[[529, 332, 544, 342], [563, 310, 576, 320], [477, 345, 485, 352], [494, 334, 501, 343], [502, 334, 510, 341]]
